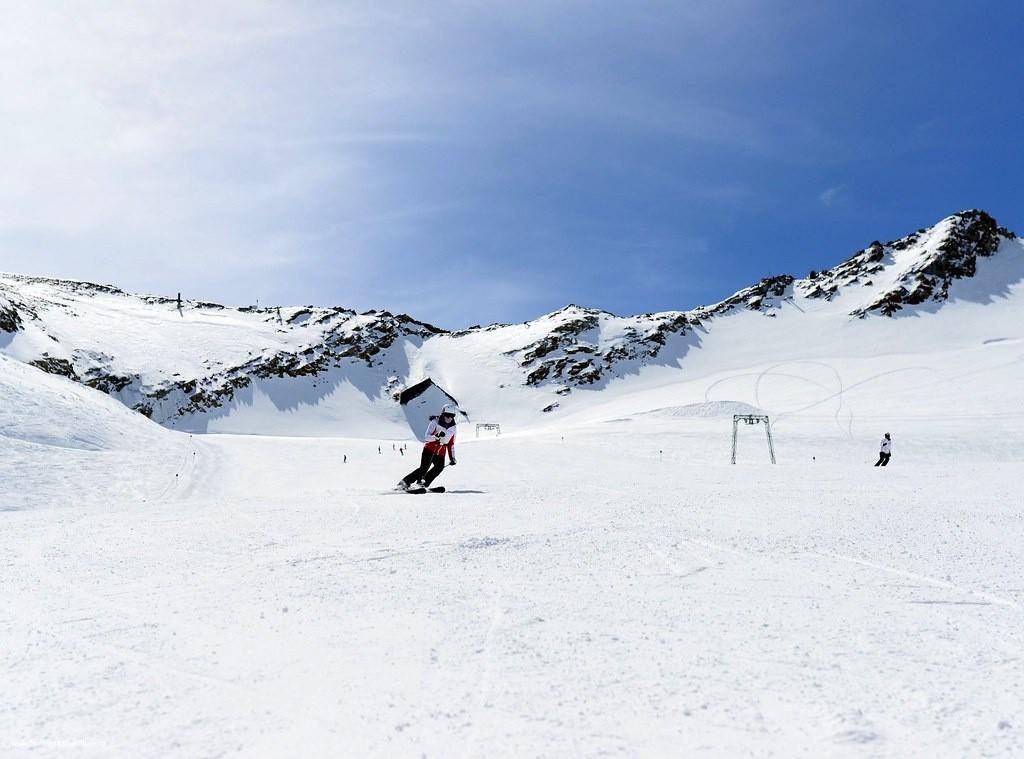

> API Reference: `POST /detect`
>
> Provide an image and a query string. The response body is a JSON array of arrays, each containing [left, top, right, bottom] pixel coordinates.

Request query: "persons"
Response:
[[875, 432, 891, 466], [394, 405, 457, 492]]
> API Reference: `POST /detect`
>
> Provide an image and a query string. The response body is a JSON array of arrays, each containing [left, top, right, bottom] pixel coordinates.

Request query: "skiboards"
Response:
[[346, 486, 446, 495]]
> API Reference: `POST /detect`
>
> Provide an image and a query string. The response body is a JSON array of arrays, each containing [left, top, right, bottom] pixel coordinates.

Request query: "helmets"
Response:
[[885, 432, 890, 436], [441, 404, 456, 417]]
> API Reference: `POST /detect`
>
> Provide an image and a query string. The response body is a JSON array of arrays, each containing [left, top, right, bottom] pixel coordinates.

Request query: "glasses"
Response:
[[446, 415, 453, 418]]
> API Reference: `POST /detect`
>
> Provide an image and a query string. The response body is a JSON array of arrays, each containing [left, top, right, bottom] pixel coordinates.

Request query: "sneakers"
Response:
[[395, 480, 407, 490], [416, 480, 426, 488]]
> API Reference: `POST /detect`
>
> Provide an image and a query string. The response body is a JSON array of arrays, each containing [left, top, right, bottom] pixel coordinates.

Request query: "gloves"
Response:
[[883, 443, 887, 446], [450, 459, 457, 465], [435, 431, 445, 438]]
